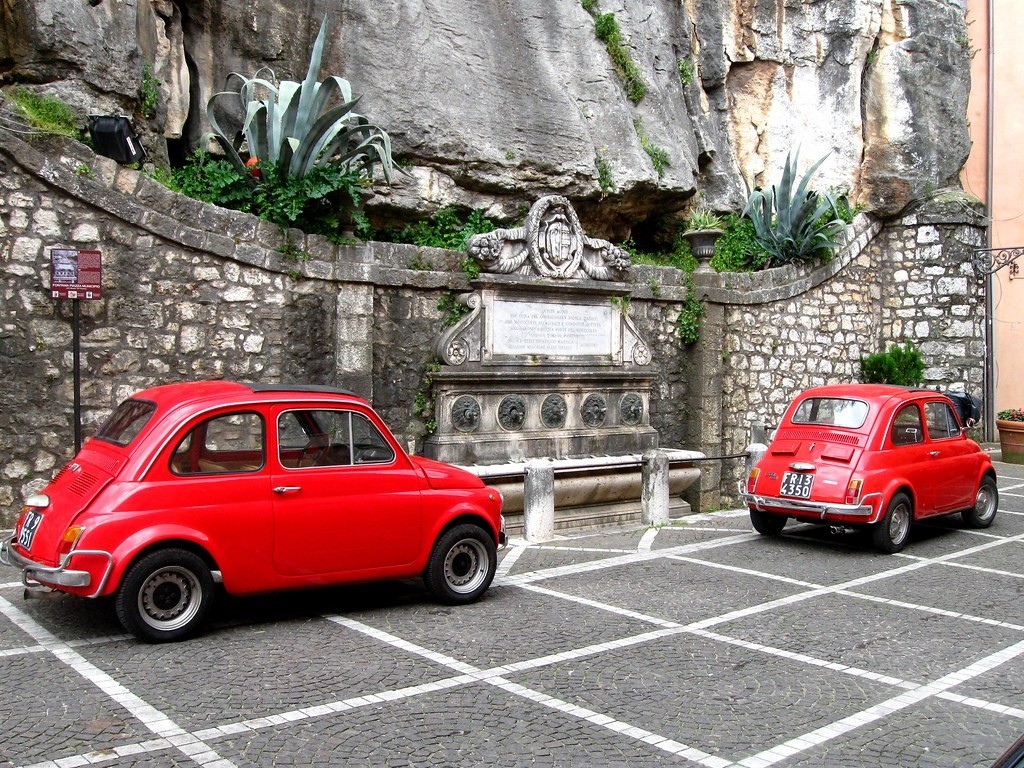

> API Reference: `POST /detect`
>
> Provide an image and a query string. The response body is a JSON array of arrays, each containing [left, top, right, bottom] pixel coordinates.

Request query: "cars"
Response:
[[737, 383, 1000, 555], [0, 378, 510, 644]]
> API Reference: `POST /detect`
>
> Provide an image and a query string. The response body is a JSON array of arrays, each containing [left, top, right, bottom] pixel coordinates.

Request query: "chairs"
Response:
[[198, 459, 230, 472]]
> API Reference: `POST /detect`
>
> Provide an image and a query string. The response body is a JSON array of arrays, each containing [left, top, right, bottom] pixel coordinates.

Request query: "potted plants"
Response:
[[682, 207, 724, 274]]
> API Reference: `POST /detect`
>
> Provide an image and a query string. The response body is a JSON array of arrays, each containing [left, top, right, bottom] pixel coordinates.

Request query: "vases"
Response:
[[995, 418, 1024, 465]]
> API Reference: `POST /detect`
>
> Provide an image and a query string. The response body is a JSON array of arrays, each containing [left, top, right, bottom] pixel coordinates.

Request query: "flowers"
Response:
[[997, 407, 1024, 422]]
